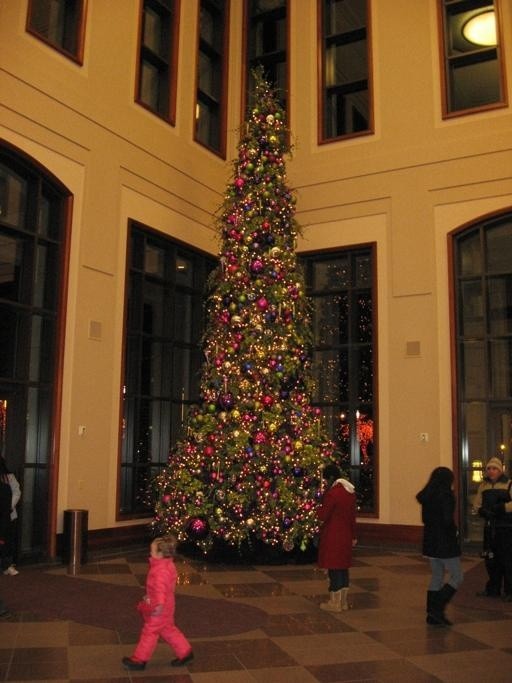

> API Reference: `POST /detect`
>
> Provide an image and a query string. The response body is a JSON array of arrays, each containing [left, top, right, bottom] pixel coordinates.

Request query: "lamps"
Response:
[[461, 7, 498, 45]]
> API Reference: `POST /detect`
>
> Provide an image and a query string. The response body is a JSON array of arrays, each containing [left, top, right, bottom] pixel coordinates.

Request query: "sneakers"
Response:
[[3, 567, 18, 575], [122, 656, 145, 670], [172, 652, 192, 665]]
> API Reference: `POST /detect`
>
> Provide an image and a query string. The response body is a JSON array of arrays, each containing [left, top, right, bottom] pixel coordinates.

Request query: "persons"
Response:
[[470, 455, 511, 602], [415, 466, 464, 626], [313, 463, 359, 612], [1, 463, 24, 576], [121, 533, 195, 670], [0, 468, 13, 575]]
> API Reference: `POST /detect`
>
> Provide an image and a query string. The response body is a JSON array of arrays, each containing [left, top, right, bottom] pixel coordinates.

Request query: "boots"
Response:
[[319, 588, 348, 612], [426, 584, 457, 627]]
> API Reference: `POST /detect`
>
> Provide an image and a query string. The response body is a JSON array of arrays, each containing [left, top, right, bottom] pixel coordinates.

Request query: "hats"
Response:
[[485, 457, 503, 471]]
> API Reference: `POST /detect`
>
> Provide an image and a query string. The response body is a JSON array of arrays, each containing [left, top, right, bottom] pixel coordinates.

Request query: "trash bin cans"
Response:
[[63, 509, 88, 566]]
